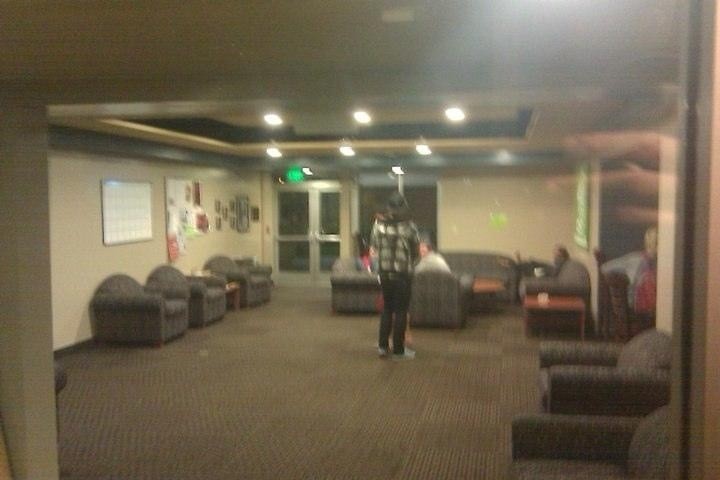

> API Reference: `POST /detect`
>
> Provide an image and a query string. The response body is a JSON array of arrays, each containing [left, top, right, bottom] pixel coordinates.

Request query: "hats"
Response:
[[387, 190, 404, 207]]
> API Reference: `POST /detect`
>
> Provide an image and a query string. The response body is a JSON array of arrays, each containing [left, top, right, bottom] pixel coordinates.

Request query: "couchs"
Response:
[[446, 252, 519, 305]]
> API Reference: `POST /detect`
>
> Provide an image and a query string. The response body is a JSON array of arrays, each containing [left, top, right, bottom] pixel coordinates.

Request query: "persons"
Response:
[[364, 244, 376, 272], [369, 190, 423, 357], [630, 220, 656, 316], [415, 241, 451, 272], [547, 246, 570, 276]]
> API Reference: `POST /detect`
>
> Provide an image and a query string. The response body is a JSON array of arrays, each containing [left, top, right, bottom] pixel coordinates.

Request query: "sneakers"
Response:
[[393, 347, 416, 360], [376, 343, 390, 357]]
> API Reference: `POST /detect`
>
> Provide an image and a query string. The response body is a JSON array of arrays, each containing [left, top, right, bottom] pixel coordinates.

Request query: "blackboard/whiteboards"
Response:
[[101, 179, 153, 246]]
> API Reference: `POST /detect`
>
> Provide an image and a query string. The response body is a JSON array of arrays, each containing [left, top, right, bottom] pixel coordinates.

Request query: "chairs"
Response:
[[505, 259, 673, 479], [331, 255, 475, 329], [92, 254, 273, 347]]
